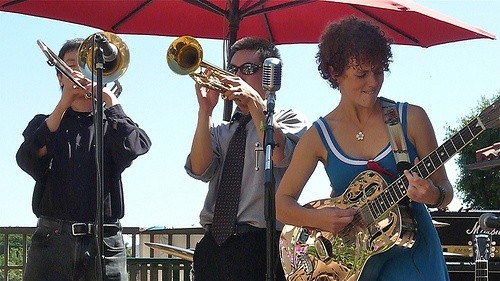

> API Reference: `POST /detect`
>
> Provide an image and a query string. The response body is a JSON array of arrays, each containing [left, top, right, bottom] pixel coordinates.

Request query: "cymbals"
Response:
[[442, 251, 463, 255], [432, 218, 450, 227]]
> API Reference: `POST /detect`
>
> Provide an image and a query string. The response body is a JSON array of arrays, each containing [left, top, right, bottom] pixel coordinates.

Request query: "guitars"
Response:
[[467, 233, 497, 281], [278, 95, 500, 281]]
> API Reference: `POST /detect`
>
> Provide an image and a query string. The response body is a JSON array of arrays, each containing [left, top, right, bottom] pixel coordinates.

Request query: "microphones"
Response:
[[94, 33, 119, 62], [479, 212, 500, 231], [262, 57, 282, 91]]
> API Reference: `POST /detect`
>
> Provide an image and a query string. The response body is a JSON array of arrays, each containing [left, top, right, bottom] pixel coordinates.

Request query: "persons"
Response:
[[184, 37, 312, 281], [276, 16, 454, 281], [15, 39, 152, 281]]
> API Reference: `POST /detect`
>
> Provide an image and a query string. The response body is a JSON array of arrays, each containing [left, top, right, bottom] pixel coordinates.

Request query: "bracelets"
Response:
[[425, 185, 446, 208]]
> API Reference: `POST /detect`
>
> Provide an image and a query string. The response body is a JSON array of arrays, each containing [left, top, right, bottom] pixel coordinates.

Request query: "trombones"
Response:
[[36, 31, 131, 108]]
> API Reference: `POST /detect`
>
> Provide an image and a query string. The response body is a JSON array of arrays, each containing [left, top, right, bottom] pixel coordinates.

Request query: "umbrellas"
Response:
[[0, 0, 498, 122]]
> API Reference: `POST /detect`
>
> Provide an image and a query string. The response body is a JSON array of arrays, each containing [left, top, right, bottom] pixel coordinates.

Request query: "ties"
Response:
[[210, 111, 268, 247]]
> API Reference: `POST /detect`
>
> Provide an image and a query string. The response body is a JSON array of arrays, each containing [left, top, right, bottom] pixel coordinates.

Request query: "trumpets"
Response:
[[166, 36, 241, 98]]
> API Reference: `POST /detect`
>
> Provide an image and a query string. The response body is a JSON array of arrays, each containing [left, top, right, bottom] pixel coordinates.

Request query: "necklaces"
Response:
[[339, 105, 374, 140]]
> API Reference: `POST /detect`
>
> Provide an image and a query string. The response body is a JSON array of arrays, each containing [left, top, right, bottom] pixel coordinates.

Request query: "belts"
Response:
[[206, 225, 258, 234], [37, 217, 121, 235]]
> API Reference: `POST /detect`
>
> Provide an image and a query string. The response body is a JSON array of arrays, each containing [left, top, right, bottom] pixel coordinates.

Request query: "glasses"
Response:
[[227, 62, 261, 75]]
